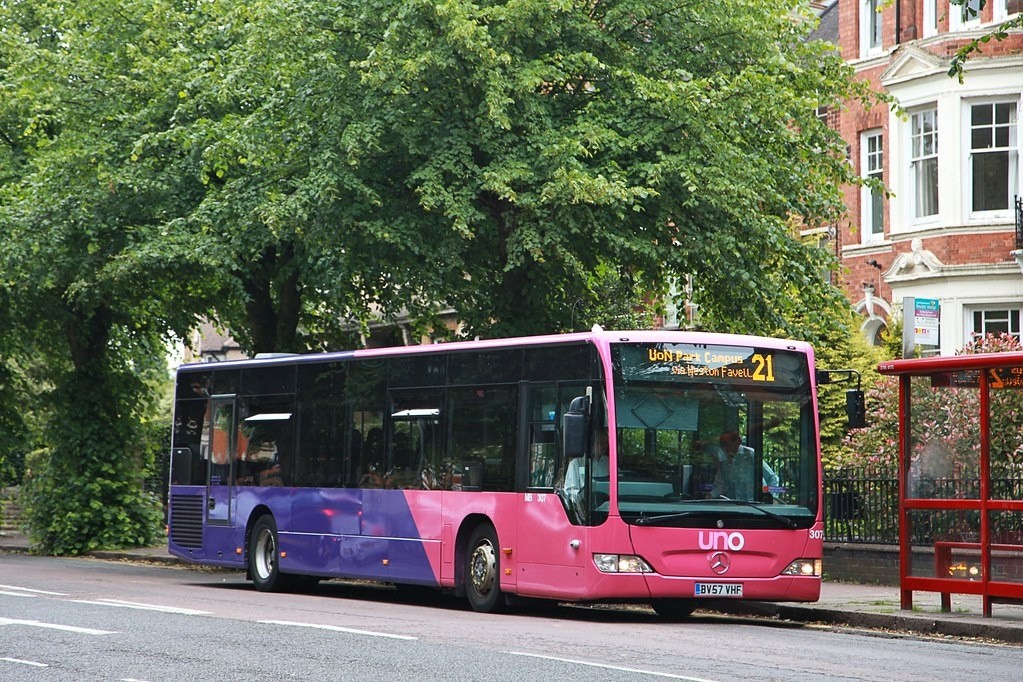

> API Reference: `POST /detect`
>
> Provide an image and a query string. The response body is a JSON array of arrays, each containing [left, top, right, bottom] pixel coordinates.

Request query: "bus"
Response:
[[166, 321, 868, 629]]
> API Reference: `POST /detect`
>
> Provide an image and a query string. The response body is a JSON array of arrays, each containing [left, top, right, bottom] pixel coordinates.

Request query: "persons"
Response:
[[563, 428, 621, 505], [702, 430, 780, 500], [352, 426, 411, 470]]
